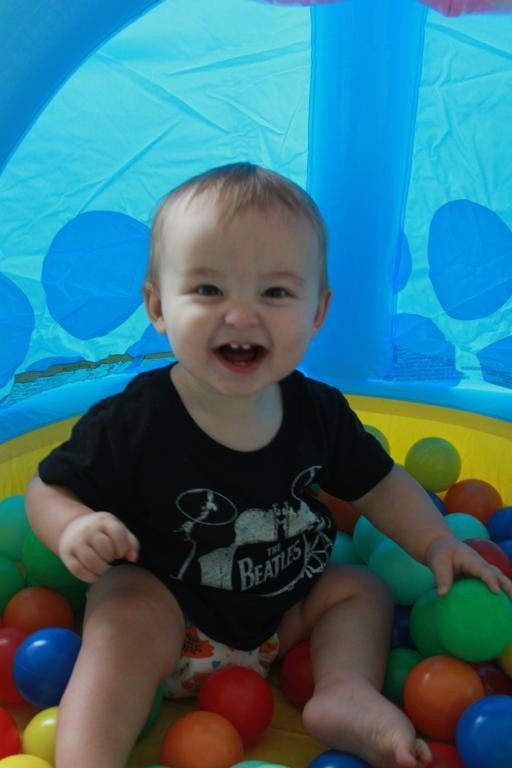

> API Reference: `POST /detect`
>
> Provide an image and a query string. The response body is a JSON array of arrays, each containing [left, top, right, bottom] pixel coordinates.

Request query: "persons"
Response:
[[24, 162, 512, 768]]
